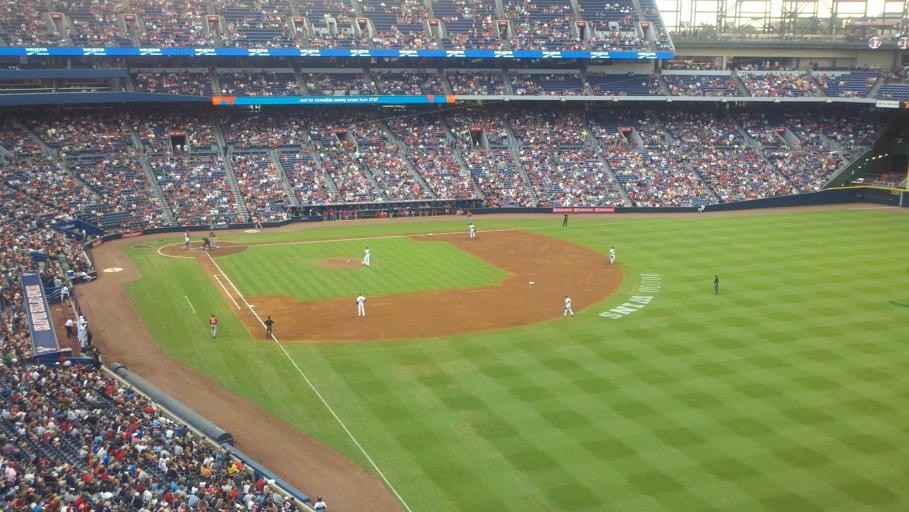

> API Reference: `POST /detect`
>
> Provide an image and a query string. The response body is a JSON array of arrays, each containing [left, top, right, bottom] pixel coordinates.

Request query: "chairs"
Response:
[[60, 437, 84, 455], [225, 7, 295, 45], [376, 0, 649, 50], [0, 418, 17, 437], [299, 2, 376, 27], [72, 205, 131, 231], [96, 391, 110, 402], [17, 440, 87, 468], [146, 463, 168, 482]]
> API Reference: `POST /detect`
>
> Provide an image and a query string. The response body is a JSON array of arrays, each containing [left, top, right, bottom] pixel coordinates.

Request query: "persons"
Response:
[[696, 102, 887, 213], [838, 80, 859, 97], [0, 366, 301, 512], [264, 316, 275, 340], [609, 246, 616, 265], [355, 293, 366, 317], [284, 103, 703, 223], [562, 295, 574, 317], [314, 496, 327, 512], [713, 275, 720, 296], [563, 213, 568, 226], [864, 63, 886, 85], [1, 228, 103, 369], [0, 105, 295, 229], [641, 57, 830, 96], [888, 63, 906, 82], [469, 223, 476, 240], [209, 314, 218, 337], [0, 0, 676, 51], [185, 229, 216, 251], [362, 245, 372, 267], [130, 66, 628, 96]]
